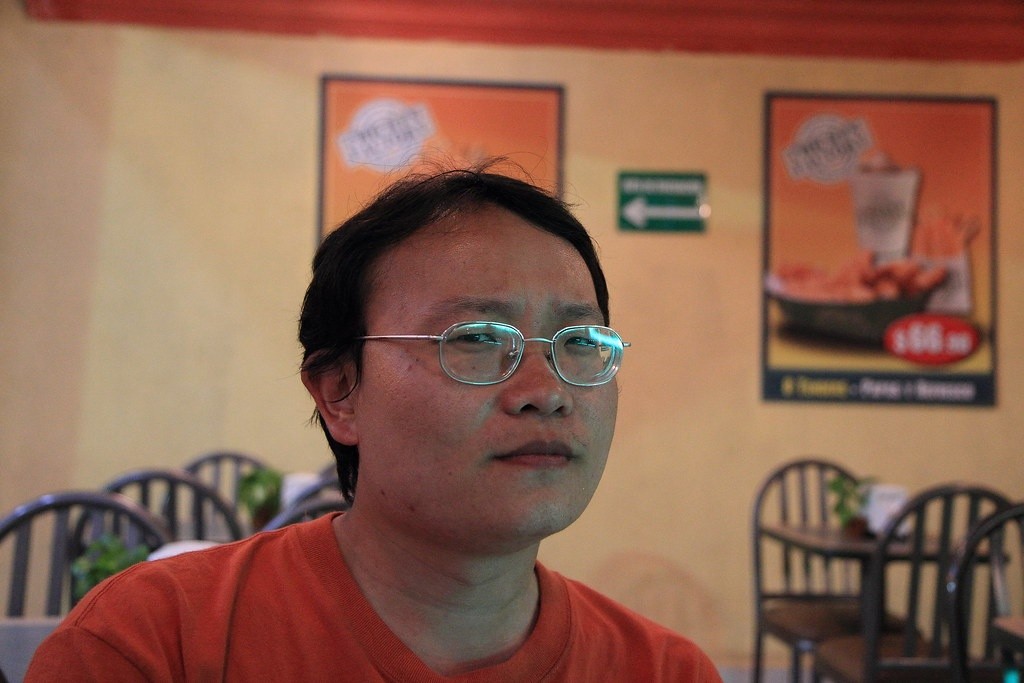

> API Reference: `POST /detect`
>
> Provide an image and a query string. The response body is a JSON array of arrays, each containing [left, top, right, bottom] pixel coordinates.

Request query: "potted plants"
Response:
[[829, 471, 881, 540], [239, 464, 282, 532]]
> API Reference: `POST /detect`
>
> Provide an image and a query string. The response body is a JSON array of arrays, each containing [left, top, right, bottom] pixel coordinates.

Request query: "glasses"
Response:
[[357, 318, 633, 389]]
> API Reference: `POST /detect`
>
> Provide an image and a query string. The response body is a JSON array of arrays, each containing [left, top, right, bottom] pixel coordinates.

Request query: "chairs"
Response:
[[862, 484, 1024, 683], [6, 451, 280, 617], [753, 459, 925, 683]]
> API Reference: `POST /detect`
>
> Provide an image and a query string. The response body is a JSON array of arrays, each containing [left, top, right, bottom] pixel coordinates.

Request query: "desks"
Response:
[[762, 524, 1011, 637]]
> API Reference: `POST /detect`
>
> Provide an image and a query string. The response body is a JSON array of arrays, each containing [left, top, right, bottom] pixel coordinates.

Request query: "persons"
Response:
[[21, 155, 723, 683]]
